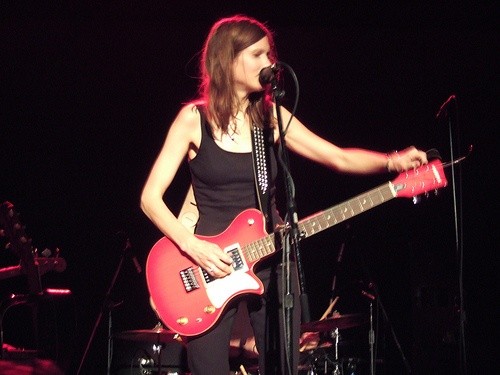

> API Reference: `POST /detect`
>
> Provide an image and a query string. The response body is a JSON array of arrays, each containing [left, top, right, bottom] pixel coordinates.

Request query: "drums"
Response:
[[109, 329, 186, 374]]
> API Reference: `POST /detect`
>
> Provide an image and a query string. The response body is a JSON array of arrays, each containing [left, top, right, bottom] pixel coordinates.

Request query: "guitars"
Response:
[[146, 145, 473, 337]]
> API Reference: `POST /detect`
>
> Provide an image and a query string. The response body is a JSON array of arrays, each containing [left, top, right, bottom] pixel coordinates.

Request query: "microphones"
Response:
[[127, 240, 142, 274], [436, 95, 455, 120], [361, 291, 376, 300], [260, 62, 280, 83]]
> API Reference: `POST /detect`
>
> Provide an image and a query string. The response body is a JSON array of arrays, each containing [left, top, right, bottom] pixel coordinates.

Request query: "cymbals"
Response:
[[301, 314, 370, 332]]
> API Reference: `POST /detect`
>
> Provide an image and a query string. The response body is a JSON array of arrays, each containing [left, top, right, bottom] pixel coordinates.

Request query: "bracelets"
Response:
[[387, 151, 402, 173]]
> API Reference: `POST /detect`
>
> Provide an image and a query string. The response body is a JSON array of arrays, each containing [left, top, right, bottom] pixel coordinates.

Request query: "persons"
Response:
[[139, 13, 430, 375]]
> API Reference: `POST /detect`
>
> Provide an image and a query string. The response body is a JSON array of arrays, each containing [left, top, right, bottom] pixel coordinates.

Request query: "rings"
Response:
[[209, 264, 216, 272]]
